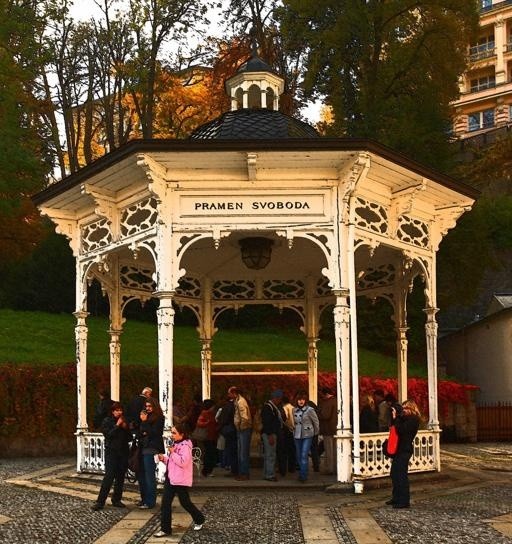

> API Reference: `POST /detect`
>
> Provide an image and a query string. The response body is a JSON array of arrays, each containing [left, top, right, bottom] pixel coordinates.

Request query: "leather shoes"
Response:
[[235, 475, 241, 480], [268, 477, 277, 481], [91, 504, 104, 510], [112, 502, 126, 508], [153, 530, 172, 537], [237, 475, 249, 481], [136, 501, 144, 505], [298, 476, 305, 482], [264, 478, 268, 480], [138, 504, 149, 509], [194, 524, 203, 531], [200, 472, 211, 481], [386, 499, 393, 505], [224, 472, 235, 477], [304, 478, 308, 480], [393, 503, 408, 508], [207, 471, 216, 477]]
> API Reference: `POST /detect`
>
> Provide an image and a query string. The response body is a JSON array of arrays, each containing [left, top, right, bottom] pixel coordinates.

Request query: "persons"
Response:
[[386, 394, 403, 425], [254, 388, 284, 481], [319, 386, 338, 476], [154, 422, 204, 537], [292, 395, 319, 482], [92, 402, 128, 510], [196, 399, 219, 480], [298, 390, 321, 473], [218, 393, 238, 476], [374, 390, 390, 432], [136, 399, 164, 509], [280, 395, 297, 476], [93, 389, 109, 432], [386, 399, 421, 509], [125, 387, 152, 428], [186, 395, 202, 433], [228, 386, 251, 481], [360, 390, 379, 434], [173, 400, 187, 425]]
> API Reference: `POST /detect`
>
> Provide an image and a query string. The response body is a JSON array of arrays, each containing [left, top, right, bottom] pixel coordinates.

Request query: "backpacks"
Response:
[[254, 404, 264, 433]]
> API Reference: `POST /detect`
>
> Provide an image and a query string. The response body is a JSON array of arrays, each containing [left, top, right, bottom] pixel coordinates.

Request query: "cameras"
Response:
[[390, 404, 404, 415]]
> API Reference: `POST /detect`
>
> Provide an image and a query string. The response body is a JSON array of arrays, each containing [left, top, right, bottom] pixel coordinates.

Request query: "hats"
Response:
[[271, 389, 283, 397]]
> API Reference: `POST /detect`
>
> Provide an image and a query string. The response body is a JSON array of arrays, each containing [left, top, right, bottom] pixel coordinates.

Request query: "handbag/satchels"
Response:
[[128, 434, 143, 472], [387, 425, 399, 454], [382, 439, 395, 458], [192, 414, 208, 441]]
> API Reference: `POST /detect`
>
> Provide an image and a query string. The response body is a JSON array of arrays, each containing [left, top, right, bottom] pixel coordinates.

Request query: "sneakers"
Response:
[[314, 464, 320, 472]]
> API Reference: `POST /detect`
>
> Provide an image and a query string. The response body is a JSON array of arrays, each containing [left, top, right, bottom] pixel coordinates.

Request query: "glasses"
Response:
[[401, 406, 411, 410]]
